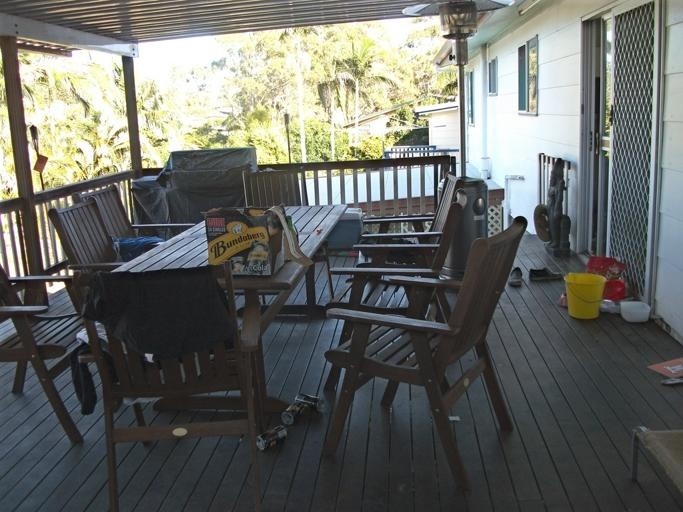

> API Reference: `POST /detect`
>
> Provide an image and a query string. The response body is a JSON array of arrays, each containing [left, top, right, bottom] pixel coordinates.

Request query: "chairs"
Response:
[[321, 215, 528, 493], [0, 185, 164, 393], [325, 188, 467, 401], [66, 260, 271, 511], [242, 171, 338, 303], [328, 174, 467, 329]]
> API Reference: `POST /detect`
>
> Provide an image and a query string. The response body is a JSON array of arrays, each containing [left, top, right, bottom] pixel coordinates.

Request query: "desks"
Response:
[[107, 205, 346, 414]]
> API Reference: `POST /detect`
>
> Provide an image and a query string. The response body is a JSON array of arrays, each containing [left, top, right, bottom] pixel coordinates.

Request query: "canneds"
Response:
[[295, 392, 320, 412], [281, 402, 307, 427], [256, 425, 288, 452], [240, 240, 270, 275]]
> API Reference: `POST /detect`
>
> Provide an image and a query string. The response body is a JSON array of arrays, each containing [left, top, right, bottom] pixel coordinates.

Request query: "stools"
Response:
[[630, 424, 682, 511]]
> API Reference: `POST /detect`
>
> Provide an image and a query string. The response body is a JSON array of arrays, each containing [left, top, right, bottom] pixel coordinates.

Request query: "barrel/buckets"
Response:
[[563, 272, 609, 321]]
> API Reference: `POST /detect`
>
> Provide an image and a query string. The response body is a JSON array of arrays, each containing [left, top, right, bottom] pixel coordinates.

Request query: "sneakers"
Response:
[[531, 267, 561, 281], [507, 267, 521, 286]]
